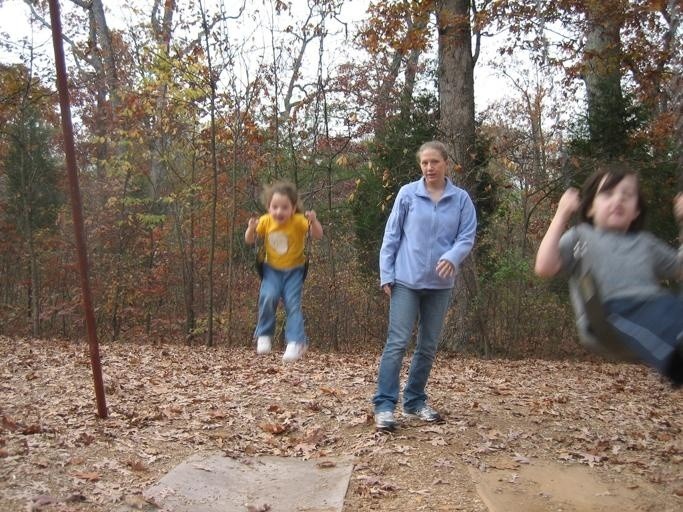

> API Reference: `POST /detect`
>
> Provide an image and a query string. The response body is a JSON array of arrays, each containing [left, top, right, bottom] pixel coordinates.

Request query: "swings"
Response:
[[535, 0, 683, 362], [219, 0, 327, 283]]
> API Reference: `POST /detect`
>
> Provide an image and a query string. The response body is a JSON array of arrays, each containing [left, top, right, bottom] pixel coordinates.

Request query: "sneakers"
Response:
[[282, 340, 305, 362], [402, 403, 439, 422], [257, 334, 271, 354], [373, 410, 397, 429]]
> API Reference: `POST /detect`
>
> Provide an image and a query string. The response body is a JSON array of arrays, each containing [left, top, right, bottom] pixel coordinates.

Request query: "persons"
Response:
[[245, 183, 323, 361], [371, 141, 477, 429], [534, 166, 683, 386]]
